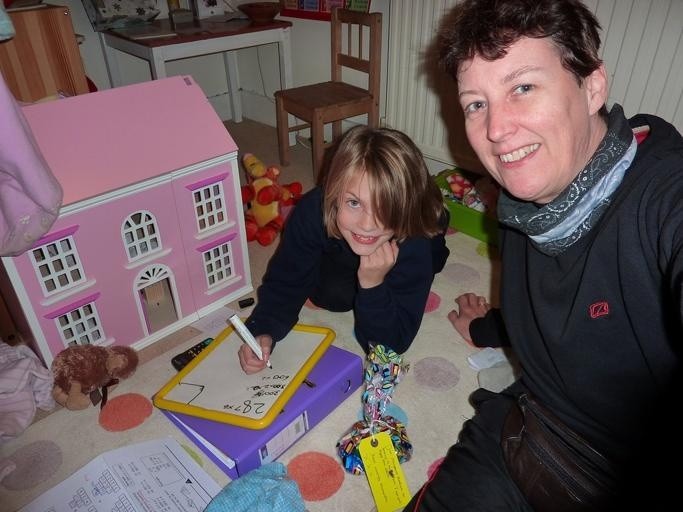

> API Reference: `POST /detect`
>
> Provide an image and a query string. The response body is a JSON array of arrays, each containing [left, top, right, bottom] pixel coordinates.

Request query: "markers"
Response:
[[230, 314, 273, 369]]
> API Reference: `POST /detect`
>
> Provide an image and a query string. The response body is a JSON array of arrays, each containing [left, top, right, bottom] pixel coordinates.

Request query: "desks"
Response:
[[96, 14, 298, 147]]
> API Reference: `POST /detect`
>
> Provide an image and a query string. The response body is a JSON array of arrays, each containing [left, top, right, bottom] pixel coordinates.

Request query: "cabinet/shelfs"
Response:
[[0, 0, 92, 103]]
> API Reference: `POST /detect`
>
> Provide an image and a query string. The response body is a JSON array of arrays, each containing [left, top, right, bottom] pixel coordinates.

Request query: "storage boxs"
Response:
[[436, 167, 499, 246]]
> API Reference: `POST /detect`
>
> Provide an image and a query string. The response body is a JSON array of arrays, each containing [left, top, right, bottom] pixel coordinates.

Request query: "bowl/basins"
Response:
[[236, 1, 284, 25]]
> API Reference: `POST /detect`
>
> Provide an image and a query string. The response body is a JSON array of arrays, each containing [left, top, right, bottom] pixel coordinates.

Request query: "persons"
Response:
[[237, 122, 450, 374], [400, 0, 681, 510]]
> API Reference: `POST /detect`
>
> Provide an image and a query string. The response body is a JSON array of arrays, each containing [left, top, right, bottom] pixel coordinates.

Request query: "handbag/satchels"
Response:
[[503, 394, 627, 511]]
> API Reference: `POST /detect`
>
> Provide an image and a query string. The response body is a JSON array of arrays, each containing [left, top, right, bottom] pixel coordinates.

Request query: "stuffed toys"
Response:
[[240, 149, 301, 246], [49, 342, 138, 410]]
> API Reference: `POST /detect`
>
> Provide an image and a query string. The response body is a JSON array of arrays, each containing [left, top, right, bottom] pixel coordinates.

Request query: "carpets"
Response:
[[0, 224, 511, 512]]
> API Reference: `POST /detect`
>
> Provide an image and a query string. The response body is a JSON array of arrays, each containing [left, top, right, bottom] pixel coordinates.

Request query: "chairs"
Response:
[[272, 7, 382, 186]]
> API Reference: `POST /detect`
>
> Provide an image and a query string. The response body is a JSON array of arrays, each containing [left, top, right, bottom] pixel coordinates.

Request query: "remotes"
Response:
[[171, 338, 215, 371]]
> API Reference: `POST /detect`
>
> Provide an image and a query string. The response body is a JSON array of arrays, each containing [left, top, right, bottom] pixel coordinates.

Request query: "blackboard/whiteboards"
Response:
[[153, 316, 336, 430]]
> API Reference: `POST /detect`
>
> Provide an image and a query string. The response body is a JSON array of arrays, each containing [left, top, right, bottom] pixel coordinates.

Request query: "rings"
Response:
[[478, 303, 485, 305]]
